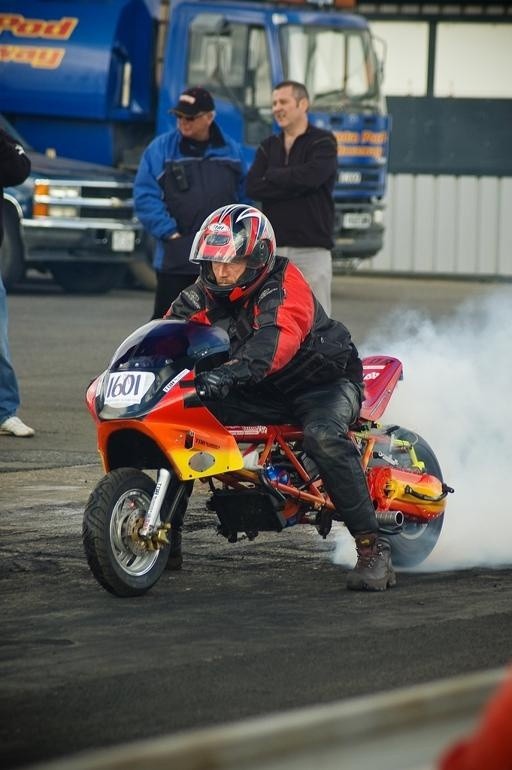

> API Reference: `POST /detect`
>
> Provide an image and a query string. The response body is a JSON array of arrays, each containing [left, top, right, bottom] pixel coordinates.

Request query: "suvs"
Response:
[[0, 112, 147, 294]]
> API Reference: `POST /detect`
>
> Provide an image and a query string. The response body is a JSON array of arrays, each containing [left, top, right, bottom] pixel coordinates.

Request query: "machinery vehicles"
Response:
[[0, 1, 394, 294]]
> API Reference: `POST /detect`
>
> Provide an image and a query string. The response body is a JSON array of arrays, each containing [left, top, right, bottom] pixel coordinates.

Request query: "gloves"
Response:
[[197, 368, 232, 403]]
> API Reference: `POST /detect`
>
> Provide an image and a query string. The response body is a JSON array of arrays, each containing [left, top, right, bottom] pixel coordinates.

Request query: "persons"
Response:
[[246, 81, 339, 320], [0, 129, 35, 435], [160, 203, 396, 590], [136, 88, 245, 319]]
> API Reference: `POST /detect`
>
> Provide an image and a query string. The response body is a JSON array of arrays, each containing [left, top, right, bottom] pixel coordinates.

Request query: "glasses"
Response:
[[174, 112, 207, 121]]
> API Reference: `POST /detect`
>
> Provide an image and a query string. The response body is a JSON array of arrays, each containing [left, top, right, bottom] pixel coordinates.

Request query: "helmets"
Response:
[[189, 202, 278, 305]]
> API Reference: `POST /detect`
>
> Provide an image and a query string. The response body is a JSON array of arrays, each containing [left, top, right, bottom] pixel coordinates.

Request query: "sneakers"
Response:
[[346, 533, 396, 590], [0, 415, 35, 437]]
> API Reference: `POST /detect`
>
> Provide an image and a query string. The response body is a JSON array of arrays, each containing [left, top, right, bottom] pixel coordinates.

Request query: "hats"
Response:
[[167, 85, 215, 117]]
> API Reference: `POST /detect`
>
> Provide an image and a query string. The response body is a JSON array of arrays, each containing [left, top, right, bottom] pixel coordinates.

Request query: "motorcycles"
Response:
[[81, 317, 456, 599]]
